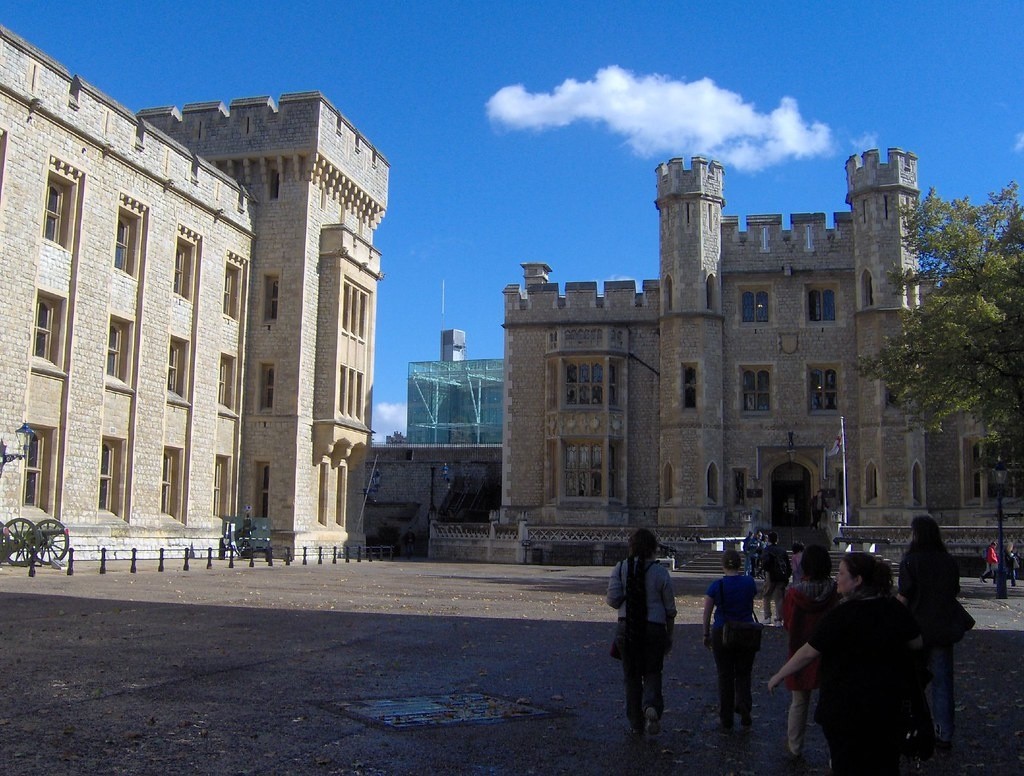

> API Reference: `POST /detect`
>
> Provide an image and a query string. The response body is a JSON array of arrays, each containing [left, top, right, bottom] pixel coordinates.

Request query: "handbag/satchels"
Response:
[[722, 622, 764, 653], [610, 641, 621, 660], [900, 678, 935, 761]]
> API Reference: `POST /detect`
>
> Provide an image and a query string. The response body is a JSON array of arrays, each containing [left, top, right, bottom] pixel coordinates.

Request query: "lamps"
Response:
[[363, 469, 381, 499], [441, 463, 450, 489], [2, 420, 35, 464]]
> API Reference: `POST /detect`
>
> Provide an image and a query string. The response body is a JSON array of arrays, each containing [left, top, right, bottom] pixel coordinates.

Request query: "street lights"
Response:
[[991, 459, 1009, 599]]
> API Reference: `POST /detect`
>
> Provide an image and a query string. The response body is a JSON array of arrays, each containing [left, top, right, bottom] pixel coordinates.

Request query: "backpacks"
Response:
[[1008, 553, 1020, 569], [765, 546, 789, 586], [982, 547, 990, 559]]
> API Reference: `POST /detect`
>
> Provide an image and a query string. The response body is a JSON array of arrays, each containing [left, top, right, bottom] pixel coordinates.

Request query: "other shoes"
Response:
[[740, 725, 751, 734], [775, 618, 782, 622], [643, 705, 660, 735], [980, 576, 987, 583], [716, 717, 729, 734], [935, 739, 953, 752], [629, 727, 641, 734], [765, 617, 772, 624]]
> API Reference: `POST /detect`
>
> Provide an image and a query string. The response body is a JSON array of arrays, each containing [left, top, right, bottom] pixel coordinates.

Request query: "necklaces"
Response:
[[704, 633, 710, 636]]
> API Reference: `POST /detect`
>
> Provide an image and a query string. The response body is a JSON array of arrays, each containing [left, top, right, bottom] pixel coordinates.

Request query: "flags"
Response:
[[828, 430, 842, 457]]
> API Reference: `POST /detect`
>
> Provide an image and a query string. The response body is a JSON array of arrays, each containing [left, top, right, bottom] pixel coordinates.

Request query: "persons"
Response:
[[809, 491, 827, 530], [703, 550, 757, 736], [767, 544, 923, 776], [896, 515, 975, 750], [404, 527, 415, 559], [743, 531, 806, 627], [980, 541, 1021, 586], [606, 528, 677, 735]]
